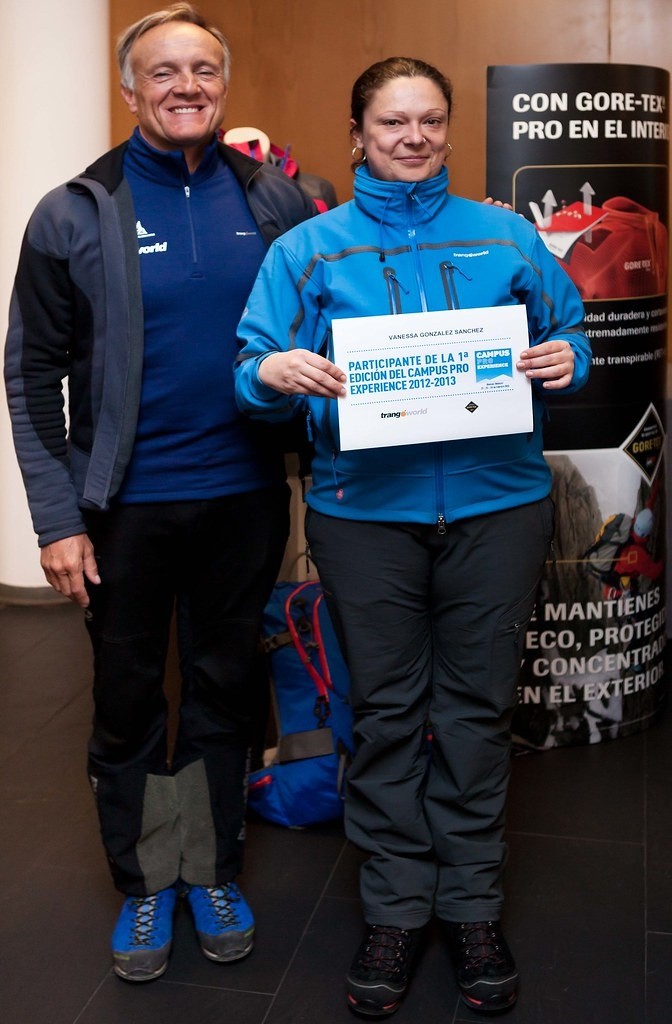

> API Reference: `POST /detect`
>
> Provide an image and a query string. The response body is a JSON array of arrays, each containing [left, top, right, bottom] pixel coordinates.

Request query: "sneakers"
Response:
[[436, 916, 521, 1012], [346, 916, 434, 1016], [180, 877, 256, 963], [111, 880, 175, 983]]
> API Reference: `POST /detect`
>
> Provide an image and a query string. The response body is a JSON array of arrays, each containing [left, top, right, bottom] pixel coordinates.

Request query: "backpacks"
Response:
[[585, 512, 633, 583], [243, 580, 356, 834]]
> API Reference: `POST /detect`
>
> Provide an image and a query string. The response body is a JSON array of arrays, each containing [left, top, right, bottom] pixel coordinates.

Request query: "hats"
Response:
[[634, 509, 653, 537]]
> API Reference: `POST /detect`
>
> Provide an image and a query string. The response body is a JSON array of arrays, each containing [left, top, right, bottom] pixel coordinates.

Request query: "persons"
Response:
[[0, 0, 526, 983], [601, 469, 666, 673], [231, 55, 593, 1024]]
